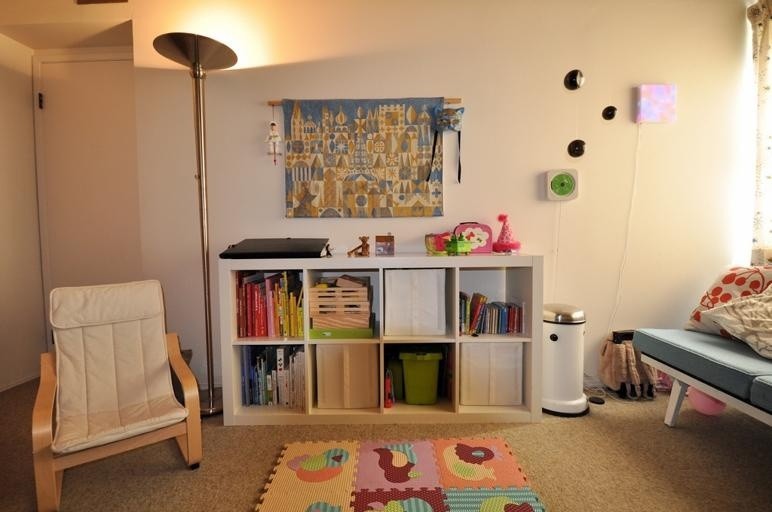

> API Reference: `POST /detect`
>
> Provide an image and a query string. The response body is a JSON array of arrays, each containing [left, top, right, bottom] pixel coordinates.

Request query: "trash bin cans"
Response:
[[543, 302, 590, 417]]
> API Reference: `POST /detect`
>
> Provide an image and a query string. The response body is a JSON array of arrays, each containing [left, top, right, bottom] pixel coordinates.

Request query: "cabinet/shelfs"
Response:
[[213, 251, 544, 424]]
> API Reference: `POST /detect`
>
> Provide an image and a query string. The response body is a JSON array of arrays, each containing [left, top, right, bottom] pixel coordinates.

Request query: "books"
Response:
[[235, 272, 303, 338], [460, 291, 522, 334], [240, 347, 305, 411]]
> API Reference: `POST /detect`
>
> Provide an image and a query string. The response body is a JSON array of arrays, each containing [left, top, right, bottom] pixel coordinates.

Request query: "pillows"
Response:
[[684, 265, 772, 343], [701, 283, 772, 360]]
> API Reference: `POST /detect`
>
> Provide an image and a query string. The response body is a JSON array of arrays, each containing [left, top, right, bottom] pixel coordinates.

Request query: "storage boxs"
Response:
[[460, 342, 523, 406], [316, 344, 379, 409], [384, 269, 446, 336], [399, 352, 443, 405]]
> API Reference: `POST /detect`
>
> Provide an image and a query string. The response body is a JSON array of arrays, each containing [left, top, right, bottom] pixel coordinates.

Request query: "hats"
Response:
[[493, 215, 520, 252]]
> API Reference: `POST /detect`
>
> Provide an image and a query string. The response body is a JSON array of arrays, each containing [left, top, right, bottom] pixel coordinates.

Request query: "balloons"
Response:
[[689, 389, 726, 416]]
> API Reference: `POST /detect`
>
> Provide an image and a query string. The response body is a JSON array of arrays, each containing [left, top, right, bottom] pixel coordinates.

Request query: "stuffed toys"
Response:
[[348, 236, 370, 256]]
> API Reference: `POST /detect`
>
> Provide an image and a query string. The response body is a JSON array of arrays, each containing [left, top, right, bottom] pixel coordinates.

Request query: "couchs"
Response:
[[632, 248, 772, 428]]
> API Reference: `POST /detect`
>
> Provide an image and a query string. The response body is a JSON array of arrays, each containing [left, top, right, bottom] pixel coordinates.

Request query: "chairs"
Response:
[[31, 279, 203, 512]]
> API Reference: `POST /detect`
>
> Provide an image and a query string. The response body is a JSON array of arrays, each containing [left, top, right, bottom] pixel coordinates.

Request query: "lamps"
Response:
[[635, 83, 677, 126], [152, 32, 239, 416]]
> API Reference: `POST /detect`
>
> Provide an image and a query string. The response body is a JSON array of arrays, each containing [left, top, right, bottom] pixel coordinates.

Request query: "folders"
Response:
[[217, 238, 333, 258]]
